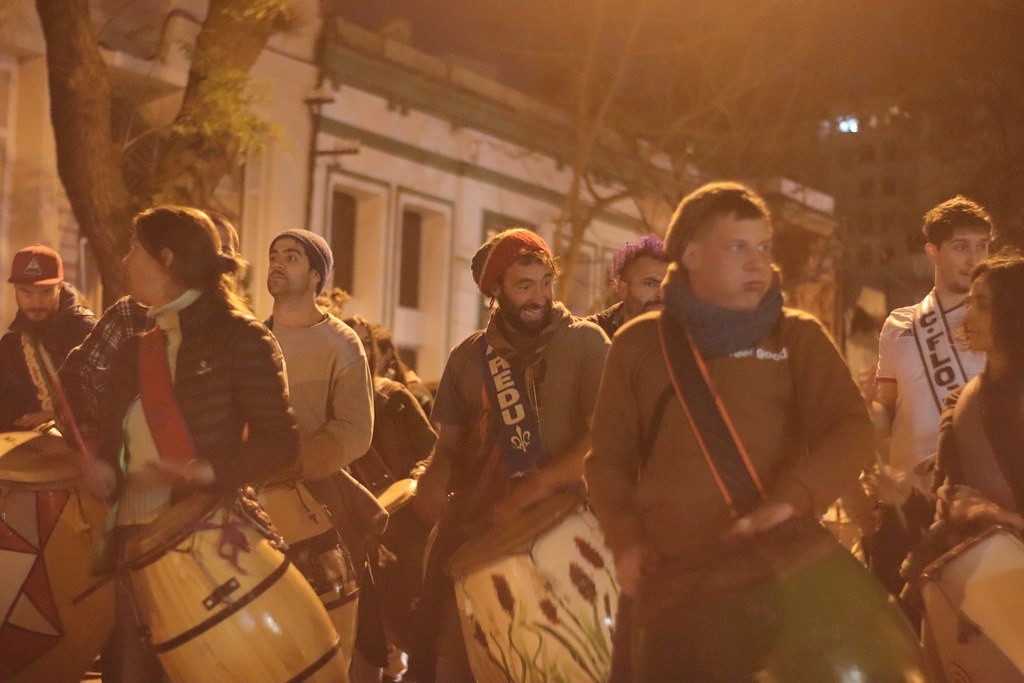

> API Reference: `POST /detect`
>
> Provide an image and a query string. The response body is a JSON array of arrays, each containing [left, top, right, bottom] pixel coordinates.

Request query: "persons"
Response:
[[413, 227, 612, 683], [900, 254, 1024, 682], [0, 246, 99, 432], [875, 193, 994, 641], [342, 317, 438, 683], [241, 226, 390, 672], [54, 204, 301, 682], [578, 232, 666, 338], [372, 323, 433, 426], [202, 210, 240, 302], [584, 181, 874, 683]]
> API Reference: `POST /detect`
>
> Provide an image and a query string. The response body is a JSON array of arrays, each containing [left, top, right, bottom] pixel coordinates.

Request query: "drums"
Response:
[[918, 522, 1024, 683], [254, 466, 360, 665], [1, 429, 117, 683], [630, 513, 937, 683], [373, 477, 435, 551], [444, 488, 620, 682], [122, 491, 349, 682]]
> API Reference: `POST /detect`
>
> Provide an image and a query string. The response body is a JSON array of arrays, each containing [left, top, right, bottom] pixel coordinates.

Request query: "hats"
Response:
[[8, 244, 64, 286], [471, 228, 551, 309], [664, 181, 756, 261], [269, 229, 333, 298]]
